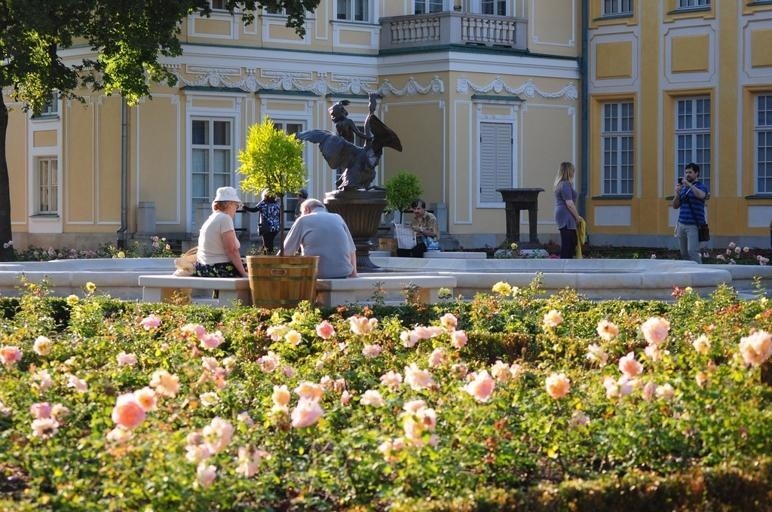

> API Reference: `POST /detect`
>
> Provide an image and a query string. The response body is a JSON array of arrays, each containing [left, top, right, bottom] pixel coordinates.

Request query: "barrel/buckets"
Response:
[[246, 256, 317, 309]]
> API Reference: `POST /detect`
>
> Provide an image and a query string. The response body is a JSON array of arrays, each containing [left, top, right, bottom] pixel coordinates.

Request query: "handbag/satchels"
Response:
[[698, 223, 710, 241], [258, 223, 268, 235]]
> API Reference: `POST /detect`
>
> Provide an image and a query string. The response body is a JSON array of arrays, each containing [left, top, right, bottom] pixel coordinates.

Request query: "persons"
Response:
[[275, 198, 359, 280], [241, 189, 280, 255], [328, 100, 370, 145], [395, 199, 440, 257], [553, 162, 581, 259], [193, 186, 248, 298], [293, 189, 308, 220], [672, 163, 708, 264]]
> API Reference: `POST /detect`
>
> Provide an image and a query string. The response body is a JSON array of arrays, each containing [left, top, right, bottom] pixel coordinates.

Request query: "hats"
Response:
[[215, 186, 241, 202]]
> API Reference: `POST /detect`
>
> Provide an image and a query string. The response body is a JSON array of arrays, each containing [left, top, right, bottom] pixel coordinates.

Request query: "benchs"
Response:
[[423, 249, 488, 261]]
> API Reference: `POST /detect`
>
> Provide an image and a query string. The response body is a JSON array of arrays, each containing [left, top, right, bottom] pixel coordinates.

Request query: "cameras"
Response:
[[678, 177, 684, 185]]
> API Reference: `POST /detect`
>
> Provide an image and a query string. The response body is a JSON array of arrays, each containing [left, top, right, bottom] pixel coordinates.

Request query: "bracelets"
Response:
[[688, 182, 691, 187], [418, 228, 423, 234]]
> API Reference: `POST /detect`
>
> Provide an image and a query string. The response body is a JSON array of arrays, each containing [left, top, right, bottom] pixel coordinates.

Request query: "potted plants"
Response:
[[378, 168, 424, 258], [234, 113, 321, 310]]
